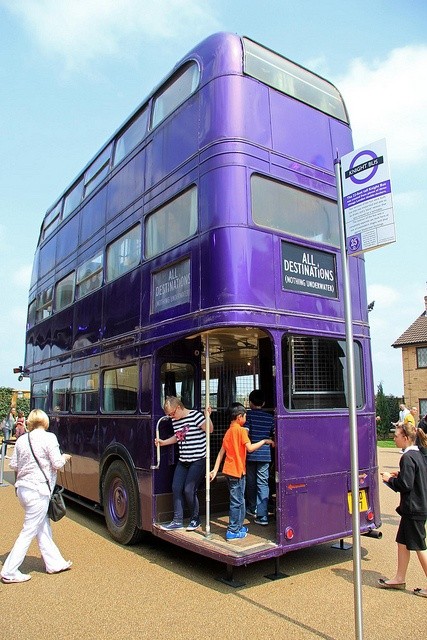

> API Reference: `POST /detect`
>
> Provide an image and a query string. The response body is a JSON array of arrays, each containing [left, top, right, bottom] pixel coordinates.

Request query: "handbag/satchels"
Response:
[[12, 427, 16, 436], [48, 484, 67, 522], [0, 420, 6, 431]]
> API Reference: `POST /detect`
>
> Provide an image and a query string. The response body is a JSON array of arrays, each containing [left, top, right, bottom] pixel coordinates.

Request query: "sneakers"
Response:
[[254, 515, 268, 525], [186, 519, 202, 531], [225, 530, 247, 541], [239, 526, 249, 533], [159, 520, 184, 531]]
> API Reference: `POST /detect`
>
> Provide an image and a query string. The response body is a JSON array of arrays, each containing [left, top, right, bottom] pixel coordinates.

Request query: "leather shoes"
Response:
[[377, 579, 406, 590], [413, 588, 427, 598]]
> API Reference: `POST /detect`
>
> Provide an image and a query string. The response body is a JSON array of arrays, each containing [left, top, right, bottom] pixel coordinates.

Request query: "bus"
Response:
[[13, 32, 382, 588]]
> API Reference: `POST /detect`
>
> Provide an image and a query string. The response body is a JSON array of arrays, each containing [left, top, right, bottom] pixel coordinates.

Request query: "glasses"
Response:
[[167, 405, 179, 418]]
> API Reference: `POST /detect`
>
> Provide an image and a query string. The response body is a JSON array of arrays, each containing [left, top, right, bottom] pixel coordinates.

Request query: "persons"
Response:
[[416, 415, 427, 447], [1, 409, 73, 582], [154, 396, 214, 530], [0, 407, 16, 459], [404, 407, 417, 427], [376, 423, 426, 598], [242, 389, 275, 525], [15, 411, 26, 438], [391, 403, 410, 454], [205, 402, 275, 541]]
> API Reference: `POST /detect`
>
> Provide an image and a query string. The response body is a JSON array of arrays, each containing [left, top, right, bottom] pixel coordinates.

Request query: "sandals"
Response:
[[47, 560, 72, 574], [2, 574, 31, 584]]
